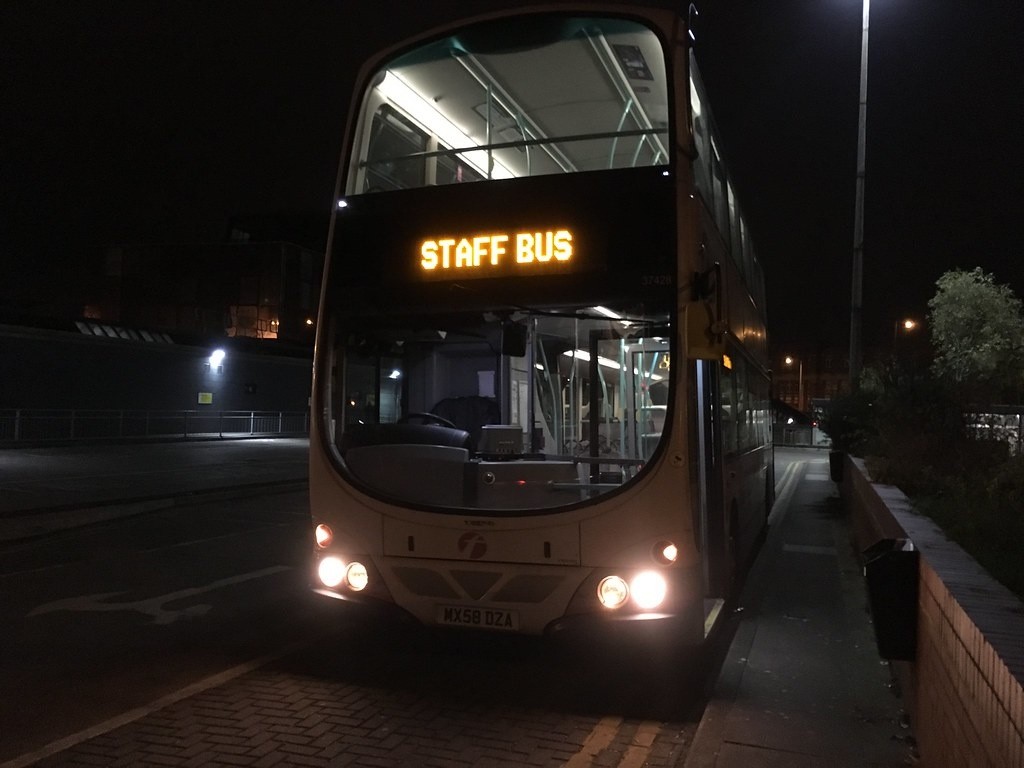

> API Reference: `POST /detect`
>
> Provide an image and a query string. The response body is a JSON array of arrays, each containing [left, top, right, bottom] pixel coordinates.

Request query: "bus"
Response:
[[307, 0, 773, 676]]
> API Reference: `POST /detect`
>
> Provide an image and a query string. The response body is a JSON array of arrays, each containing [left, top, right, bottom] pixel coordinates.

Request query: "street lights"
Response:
[[847, 0, 888, 387], [785, 357, 804, 412], [894, 319, 915, 354]]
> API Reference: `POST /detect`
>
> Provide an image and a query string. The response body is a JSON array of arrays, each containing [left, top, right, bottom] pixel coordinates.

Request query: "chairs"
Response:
[[423, 396, 501, 452]]
